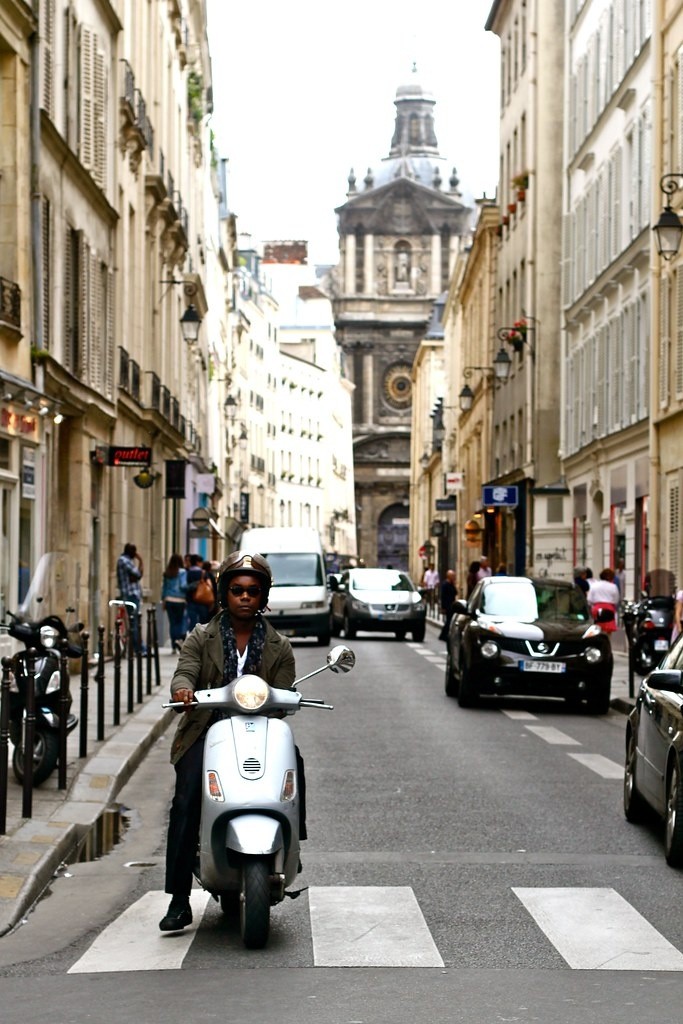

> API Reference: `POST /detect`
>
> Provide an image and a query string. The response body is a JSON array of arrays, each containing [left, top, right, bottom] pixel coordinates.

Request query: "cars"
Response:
[[623, 633, 683, 872], [445, 576, 614, 717], [326, 568, 426, 642]]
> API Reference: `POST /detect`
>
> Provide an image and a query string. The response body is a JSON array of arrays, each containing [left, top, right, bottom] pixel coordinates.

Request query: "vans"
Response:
[[236, 525, 334, 645]]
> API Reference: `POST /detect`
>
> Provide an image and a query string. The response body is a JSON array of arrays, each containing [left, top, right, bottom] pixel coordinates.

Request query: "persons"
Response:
[[467, 556, 492, 600], [495, 562, 506, 576], [420, 563, 440, 590], [158, 551, 308, 932], [573, 567, 622, 640], [162, 554, 220, 654], [439, 569, 467, 640], [116, 543, 153, 657], [671, 589, 683, 647]]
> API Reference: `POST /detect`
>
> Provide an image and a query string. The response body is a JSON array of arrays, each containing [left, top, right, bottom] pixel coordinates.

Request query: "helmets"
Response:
[[217, 550, 274, 616]]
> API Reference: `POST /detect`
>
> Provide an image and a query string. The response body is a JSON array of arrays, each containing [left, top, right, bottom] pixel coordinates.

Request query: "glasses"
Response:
[[227, 585, 262, 598]]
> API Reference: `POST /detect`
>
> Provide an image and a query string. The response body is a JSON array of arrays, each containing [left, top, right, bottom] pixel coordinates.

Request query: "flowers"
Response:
[[504, 319, 526, 351]]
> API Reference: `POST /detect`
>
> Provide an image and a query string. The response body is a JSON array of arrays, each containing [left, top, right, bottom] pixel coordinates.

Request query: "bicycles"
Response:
[[62, 601, 138, 669]]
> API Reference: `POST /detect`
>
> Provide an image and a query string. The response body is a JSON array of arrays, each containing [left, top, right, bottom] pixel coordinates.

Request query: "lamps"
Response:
[[22, 397, 34, 412], [212, 376, 238, 419], [157, 279, 201, 344], [402, 484, 420, 506], [2, 385, 12, 404], [52, 410, 64, 424], [230, 418, 248, 451], [458, 365, 494, 412], [256, 483, 265, 497], [651, 173, 683, 262], [37, 403, 48, 415], [492, 307, 535, 383], [418, 440, 435, 470], [434, 404, 458, 441]]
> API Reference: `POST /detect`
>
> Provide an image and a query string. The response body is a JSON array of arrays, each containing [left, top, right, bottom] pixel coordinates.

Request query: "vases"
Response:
[[520, 330, 527, 342], [513, 343, 523, 352]]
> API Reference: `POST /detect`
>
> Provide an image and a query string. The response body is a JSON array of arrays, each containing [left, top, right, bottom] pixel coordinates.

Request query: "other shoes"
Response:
[[141, 654, 154, 658], [159, 896, 192, 931], [175, 639, 184, 652], [171, 650, 176, 655]]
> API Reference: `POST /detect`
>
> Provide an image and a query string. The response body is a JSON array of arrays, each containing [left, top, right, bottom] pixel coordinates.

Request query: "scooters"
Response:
[[161, 646, 355, 952], [618, 589, 669, 677], [0, 598, 88, 789]]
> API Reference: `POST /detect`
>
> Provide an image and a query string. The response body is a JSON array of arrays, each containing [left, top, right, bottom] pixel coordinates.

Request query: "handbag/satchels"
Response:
[[192, 569, 215, 607]]
